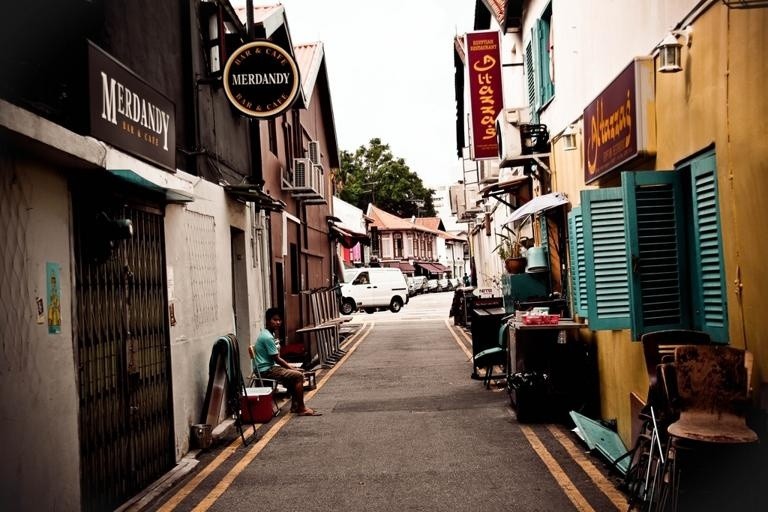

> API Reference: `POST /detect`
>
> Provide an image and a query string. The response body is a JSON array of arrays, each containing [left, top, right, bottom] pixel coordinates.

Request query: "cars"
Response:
[[407, 275, 460, 297]]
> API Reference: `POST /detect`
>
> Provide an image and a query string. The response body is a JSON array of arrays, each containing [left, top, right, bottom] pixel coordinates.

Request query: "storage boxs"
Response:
[[239, 386, 273, 423]]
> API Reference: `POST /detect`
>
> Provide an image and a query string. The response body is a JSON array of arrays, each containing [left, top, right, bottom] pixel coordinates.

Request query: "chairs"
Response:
[[245, 346, 282, 417], [610, 330, 757, 511], [475, 321, 510, 389]]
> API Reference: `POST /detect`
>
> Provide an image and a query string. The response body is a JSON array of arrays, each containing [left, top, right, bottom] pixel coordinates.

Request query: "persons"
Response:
[[48, 277, 61, 325], [252, 307, 322, 416], [360, 274, 368, 284], [369, 256, 381, 268], [463, 273, 469, 286]]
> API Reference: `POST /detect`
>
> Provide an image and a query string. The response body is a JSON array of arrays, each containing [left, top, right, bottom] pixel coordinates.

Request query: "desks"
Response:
[[507, 317, 590, 423]]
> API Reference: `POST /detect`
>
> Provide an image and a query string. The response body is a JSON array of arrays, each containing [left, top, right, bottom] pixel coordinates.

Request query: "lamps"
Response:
[[561, 119, 583, 150], [655, 24, 693, 74]]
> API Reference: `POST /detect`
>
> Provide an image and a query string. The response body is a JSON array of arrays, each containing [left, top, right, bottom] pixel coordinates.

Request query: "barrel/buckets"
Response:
[[189, 424, 212, 448]]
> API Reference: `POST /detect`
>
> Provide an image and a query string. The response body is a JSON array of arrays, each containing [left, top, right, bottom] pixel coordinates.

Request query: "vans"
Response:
[[339, 267, 409, 313]]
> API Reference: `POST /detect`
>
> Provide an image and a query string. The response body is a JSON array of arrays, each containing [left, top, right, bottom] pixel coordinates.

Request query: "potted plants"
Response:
[[497, 239, 534, 273]]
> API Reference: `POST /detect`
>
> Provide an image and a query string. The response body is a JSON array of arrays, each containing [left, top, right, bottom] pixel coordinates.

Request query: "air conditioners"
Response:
[[292, 140, 325, 200], [479, 108, 531, 181], [449, 184, 480, 219]]
